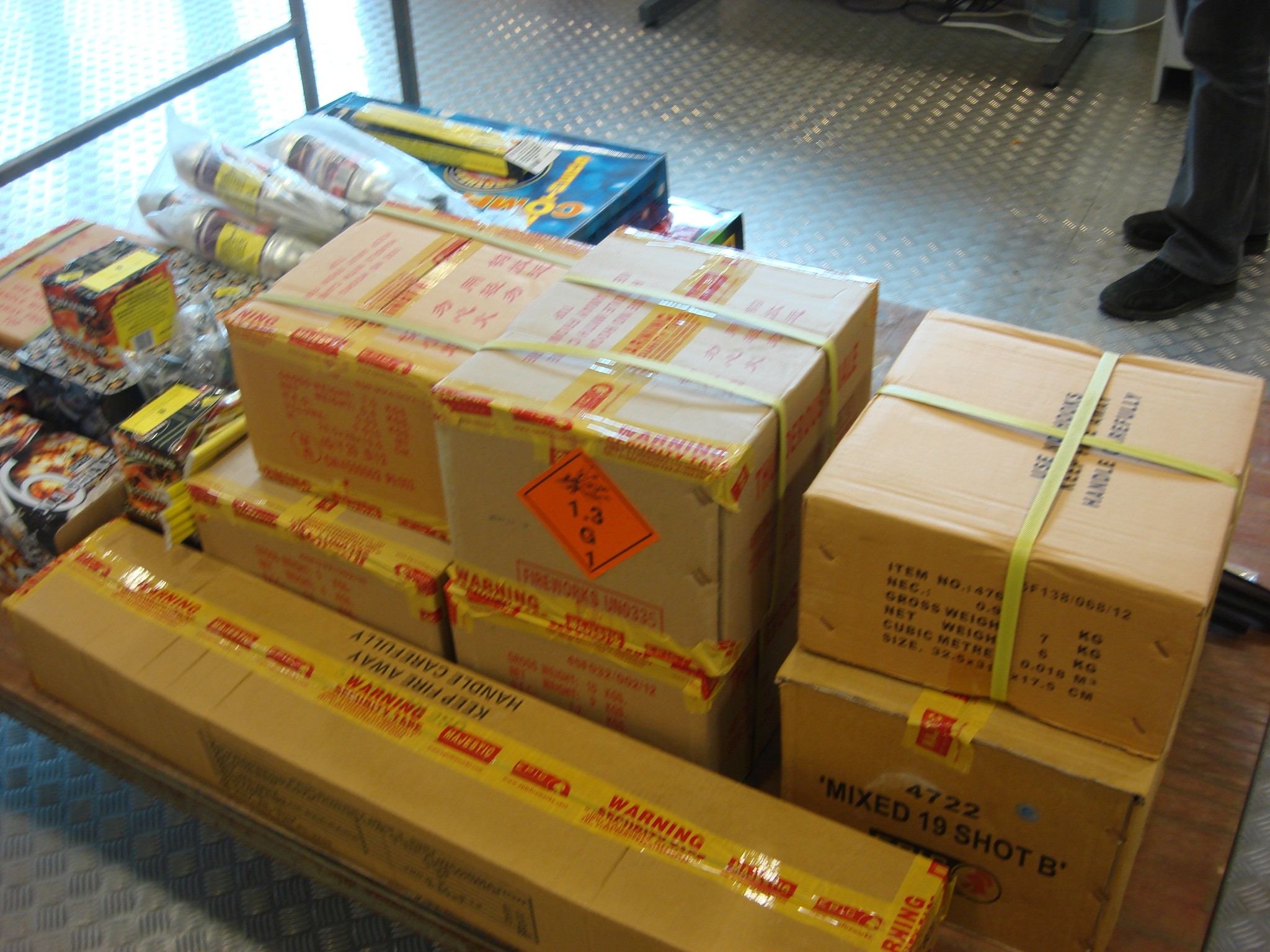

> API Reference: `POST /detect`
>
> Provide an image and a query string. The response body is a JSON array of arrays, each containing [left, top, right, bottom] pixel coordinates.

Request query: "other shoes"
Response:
[[1123, 202, 1270, 256], [1097, 255, 1239, 321]]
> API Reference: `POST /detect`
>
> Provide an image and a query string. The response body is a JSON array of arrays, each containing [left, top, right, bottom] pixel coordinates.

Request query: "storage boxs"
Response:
[[1, 88, 1269, 952]]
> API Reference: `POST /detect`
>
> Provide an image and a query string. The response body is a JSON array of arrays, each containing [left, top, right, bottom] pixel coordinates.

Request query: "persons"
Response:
[[1098, 0, 1270, 322]]
